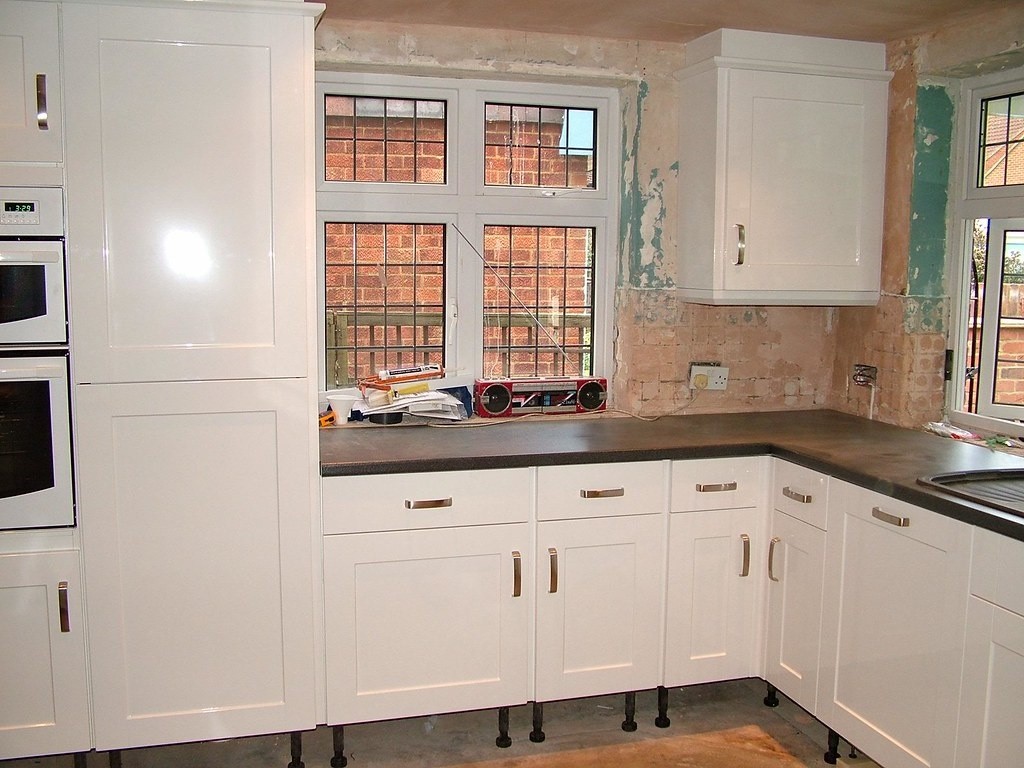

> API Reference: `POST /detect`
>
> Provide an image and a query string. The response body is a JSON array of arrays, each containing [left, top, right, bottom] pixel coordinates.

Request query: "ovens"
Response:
[[0, 186, 75, 529]]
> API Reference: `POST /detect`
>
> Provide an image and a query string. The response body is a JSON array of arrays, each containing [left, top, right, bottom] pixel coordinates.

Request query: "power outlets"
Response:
[[690, 364, 731, 392]]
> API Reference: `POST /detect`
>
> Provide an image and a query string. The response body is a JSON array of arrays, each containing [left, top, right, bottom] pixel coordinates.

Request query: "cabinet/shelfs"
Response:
[[817, 478, 1024, 768], [767, 456, 829, 706], [656, 457, 772, 729], [324, 460, 672, 743], [675, 28, 895, 306], [0, 0, 322, 768]]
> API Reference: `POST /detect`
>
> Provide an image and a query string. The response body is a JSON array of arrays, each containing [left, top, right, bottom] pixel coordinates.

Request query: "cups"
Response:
[[326, 394, 356, 425]]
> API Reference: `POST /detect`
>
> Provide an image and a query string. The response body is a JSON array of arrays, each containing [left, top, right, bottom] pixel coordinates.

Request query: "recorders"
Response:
[[451, 223, 607, 420]]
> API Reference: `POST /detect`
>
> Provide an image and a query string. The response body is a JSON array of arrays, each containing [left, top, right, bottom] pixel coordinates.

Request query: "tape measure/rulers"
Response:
[[319, 411, 335, 427]]
